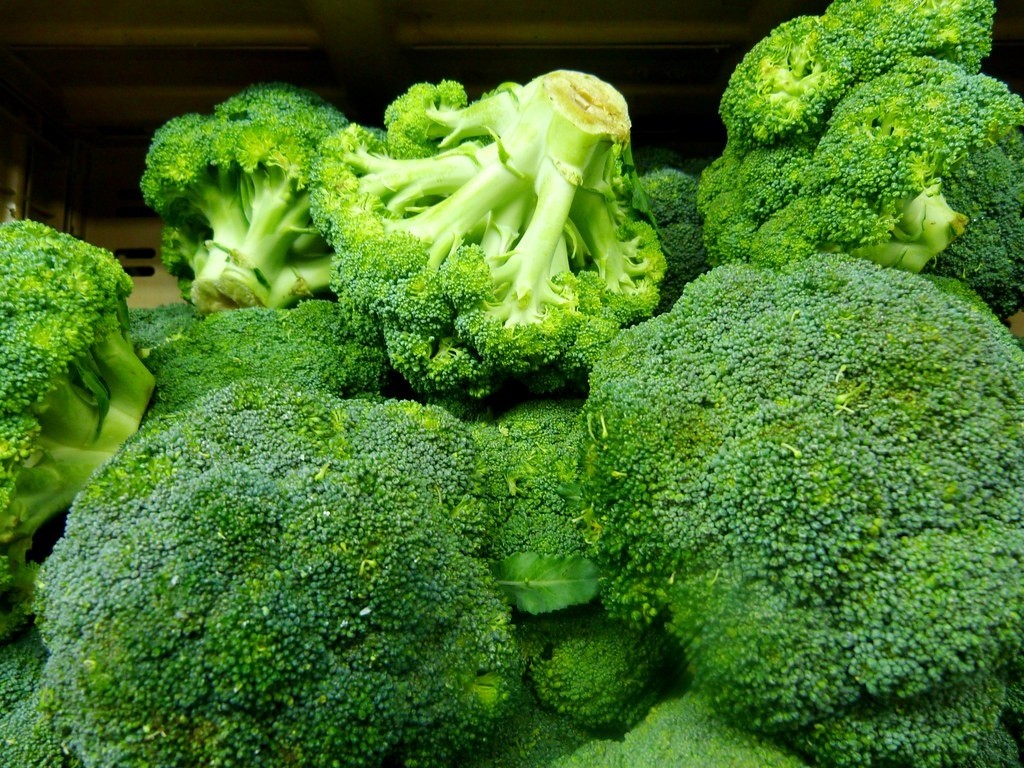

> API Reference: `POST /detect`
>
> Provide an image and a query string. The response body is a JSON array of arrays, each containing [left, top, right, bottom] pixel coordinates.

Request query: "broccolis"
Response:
[[0, 0, 1024, 768]]
